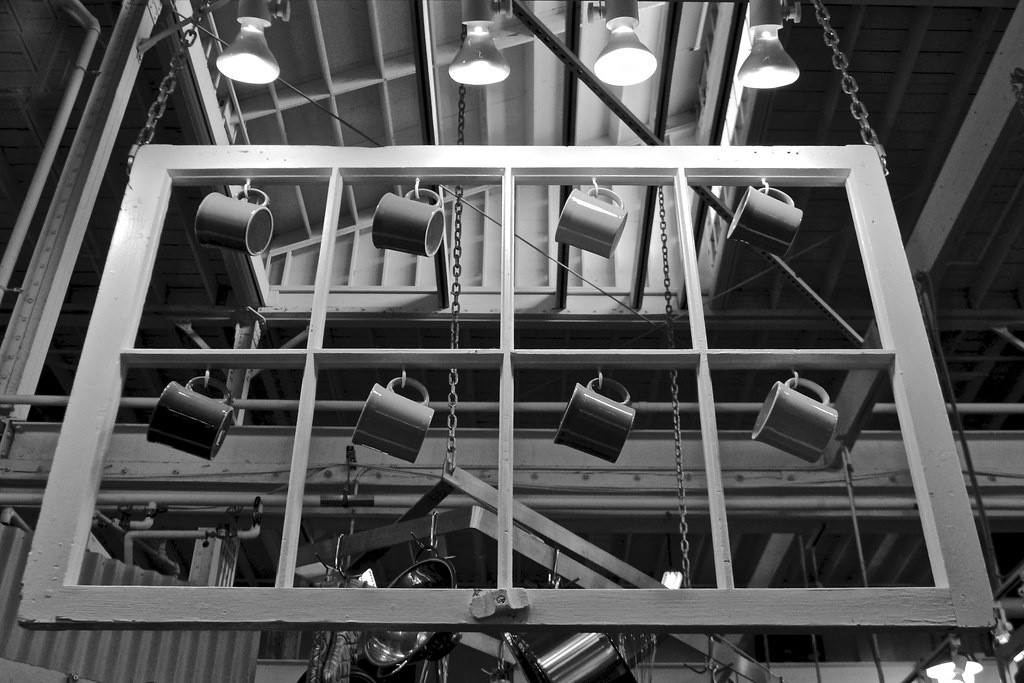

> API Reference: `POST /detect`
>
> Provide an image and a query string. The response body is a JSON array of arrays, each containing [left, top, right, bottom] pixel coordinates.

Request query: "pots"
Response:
[[361, 544, 462, 678], [506, 631, 640, 683]]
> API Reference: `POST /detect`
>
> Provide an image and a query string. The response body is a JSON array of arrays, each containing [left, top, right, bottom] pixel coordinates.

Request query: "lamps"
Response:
[[734, 0, 805, 91], [591, 0, 659, 87], [214, 0, 291, 86], [446, 0, 511, 85], [926, 641, 984, 683]]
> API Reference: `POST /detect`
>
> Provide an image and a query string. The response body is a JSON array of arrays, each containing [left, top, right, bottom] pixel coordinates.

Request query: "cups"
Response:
[[555, 187, 629, 258], [371, 188, 444, 258], [751, 375, 838, 462], [194, 186, 274, 257], [725, 185, 804, 256], [348, 375, 436, 463], [554, 378, 636, 463], [146, 373, 236, 461]]
